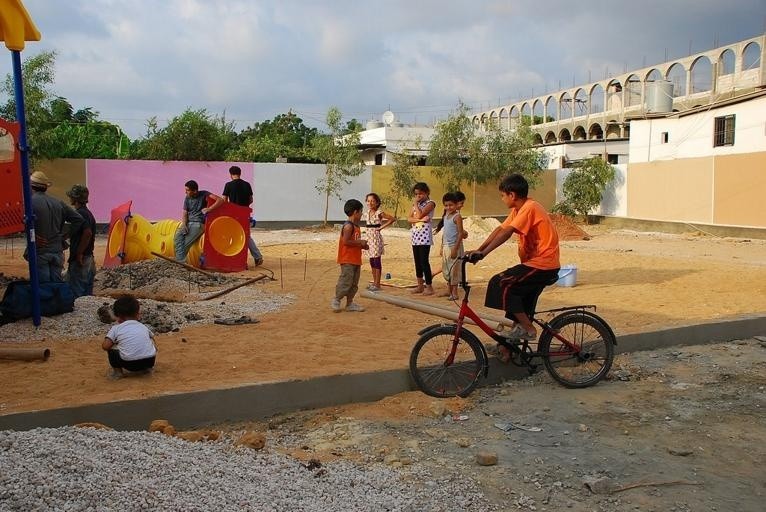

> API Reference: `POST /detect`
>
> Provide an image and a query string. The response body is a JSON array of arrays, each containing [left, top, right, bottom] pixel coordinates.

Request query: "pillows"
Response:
[[30, 171, 49, 188], [66, 183, 89, 204]]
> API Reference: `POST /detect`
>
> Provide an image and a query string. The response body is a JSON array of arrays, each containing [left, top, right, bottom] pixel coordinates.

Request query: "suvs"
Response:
[[555, 264, 578, 287]]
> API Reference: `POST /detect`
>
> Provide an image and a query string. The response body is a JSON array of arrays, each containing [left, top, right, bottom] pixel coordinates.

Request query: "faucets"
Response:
[[484, 343, 510, 364], [499, 323, 537, 339]]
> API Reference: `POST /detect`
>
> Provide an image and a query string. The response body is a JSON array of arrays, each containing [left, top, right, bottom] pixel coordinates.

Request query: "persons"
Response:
[[433, 191, 468, 284], [169, 180, 224, 262], [22, 171, 83, 280], [223, 166, 263, 266], [438, 193, 463, 301], [332, 199, 369, 312], [463, 174, 560, 355], [103, 293, 157, 379], [407, 182, 436, 295], [64, 182, 96, 297], [366, 194, 394, 290]]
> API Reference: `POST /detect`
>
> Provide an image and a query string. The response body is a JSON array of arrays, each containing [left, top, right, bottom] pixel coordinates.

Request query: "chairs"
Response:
[[345, 303, 365, 312], [448, 295, 458, 301], [332, 298, 340, 312]]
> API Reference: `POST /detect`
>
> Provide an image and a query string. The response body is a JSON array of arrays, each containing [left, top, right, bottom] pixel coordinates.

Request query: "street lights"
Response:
[[0, 281, 74, 320]]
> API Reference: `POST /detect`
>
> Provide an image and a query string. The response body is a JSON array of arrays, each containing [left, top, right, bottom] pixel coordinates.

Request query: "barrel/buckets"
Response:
[[366, 283, 383, 291]]
